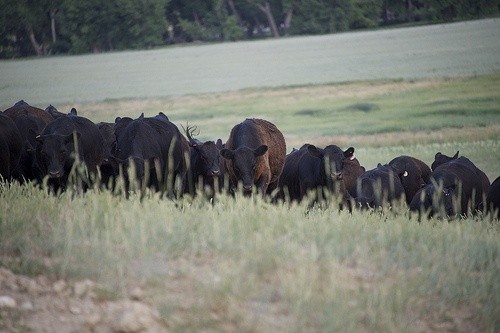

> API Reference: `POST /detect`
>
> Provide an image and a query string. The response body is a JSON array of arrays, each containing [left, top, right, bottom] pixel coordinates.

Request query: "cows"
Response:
[[0, 101, 500, 221]]
